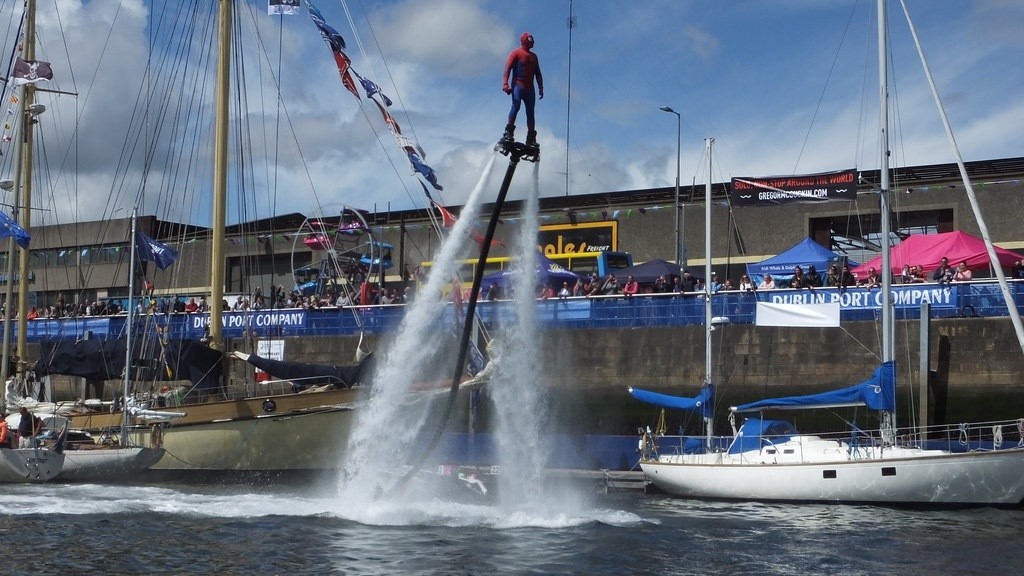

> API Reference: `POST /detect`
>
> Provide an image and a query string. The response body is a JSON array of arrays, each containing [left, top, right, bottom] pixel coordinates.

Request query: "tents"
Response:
[[746, 237, 848, 290]]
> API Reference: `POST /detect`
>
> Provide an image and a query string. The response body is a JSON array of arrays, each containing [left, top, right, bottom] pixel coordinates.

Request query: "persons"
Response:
[[0, 413, 7, 447], [503, 32, 544, 148], [740, 256, 972, 291], [642, 432, 647, 461], [2, 265, 734, 326], [17, 407, 46, 449], [1012, 259, 1024, 279]]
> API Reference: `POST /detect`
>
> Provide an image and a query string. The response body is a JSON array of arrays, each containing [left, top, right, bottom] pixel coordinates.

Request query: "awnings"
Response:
[[849, 230, 1024, 285]]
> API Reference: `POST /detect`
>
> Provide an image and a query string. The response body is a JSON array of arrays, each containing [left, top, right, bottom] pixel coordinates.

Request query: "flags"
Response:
[[0, 210, 31, 250], [115, 247, 120, 252], [133, 226, 179, 272], [268, 0, 300, 15]]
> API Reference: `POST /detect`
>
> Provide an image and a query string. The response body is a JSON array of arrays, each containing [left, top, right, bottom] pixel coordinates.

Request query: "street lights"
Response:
[[660, 105, 681, 266]]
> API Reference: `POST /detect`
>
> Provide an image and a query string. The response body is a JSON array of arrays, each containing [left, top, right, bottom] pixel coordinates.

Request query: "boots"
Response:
[[525, 130, 540, 148], [502, 123, 516, 142]]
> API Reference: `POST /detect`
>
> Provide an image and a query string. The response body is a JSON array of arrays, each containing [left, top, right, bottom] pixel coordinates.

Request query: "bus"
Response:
[[404, 251, 634, 303]]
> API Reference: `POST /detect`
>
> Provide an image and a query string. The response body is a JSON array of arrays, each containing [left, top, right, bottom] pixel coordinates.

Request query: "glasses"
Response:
[[200, 297, 205, 299], [869, 271, 875, 273], [941, 261, 947, 263], [742, 277, 748, 280]]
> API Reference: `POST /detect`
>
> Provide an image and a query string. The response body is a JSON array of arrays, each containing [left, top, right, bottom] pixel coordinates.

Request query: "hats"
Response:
[[404, 287, 412, 292], [809, 265, 816, 270]]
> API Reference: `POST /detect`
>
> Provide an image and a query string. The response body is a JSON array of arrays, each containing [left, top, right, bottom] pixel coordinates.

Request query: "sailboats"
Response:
[[0, 0, 71, 484], [625, 0, 1024, 509], [48, 0, 505, 487]]
